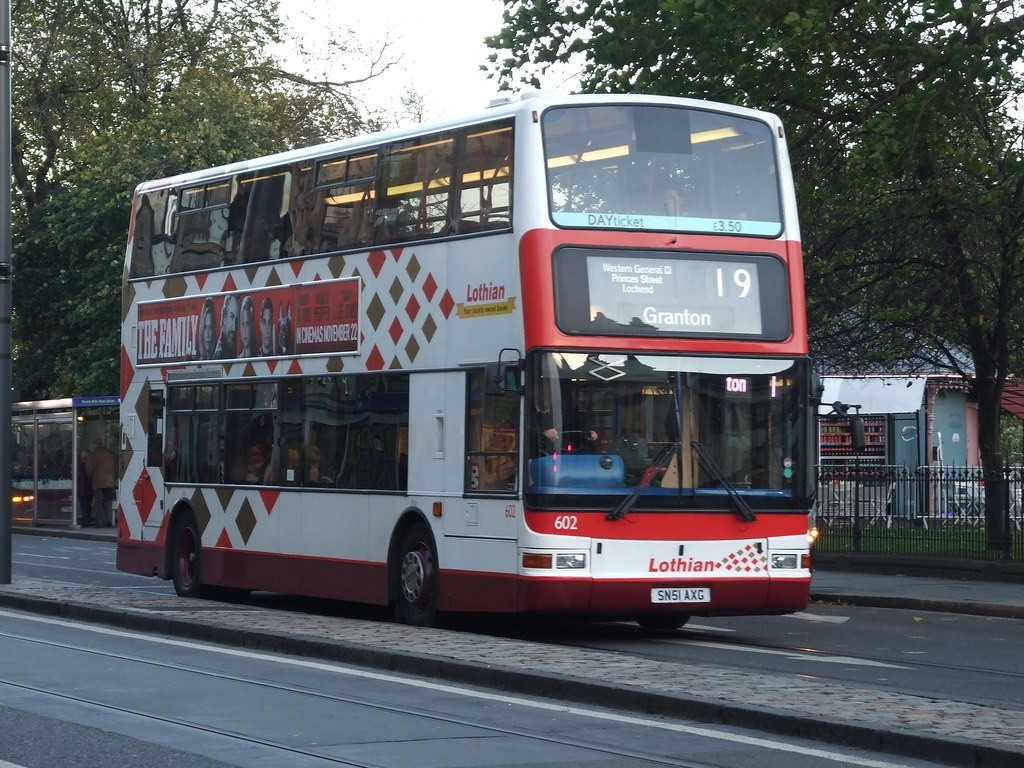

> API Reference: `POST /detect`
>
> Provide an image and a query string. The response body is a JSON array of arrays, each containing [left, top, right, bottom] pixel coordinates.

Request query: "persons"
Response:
[[534, 397, 599, 453], [257, 298, 273, 356], [86, 441, 116, 527], [286, 446, 321, 482], [215, 294, 241, 358], [78, 449, 92, 526], [238, 295, 259, 358], [198, 297, 217, 359]]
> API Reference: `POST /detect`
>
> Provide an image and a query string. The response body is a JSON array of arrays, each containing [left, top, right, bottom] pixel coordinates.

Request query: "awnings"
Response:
[[818, 375, 927, 415], [1000, 380, 1024, 418]]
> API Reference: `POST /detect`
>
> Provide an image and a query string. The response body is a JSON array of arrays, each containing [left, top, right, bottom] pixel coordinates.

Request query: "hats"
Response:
[[248, 445, 263, 455]]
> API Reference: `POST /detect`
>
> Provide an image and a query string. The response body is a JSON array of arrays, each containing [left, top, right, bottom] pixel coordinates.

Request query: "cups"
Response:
[[287, 469, 294, 482]]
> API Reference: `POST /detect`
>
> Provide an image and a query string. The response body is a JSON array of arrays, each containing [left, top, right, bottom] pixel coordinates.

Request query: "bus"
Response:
[[114, 87, 868, 638]]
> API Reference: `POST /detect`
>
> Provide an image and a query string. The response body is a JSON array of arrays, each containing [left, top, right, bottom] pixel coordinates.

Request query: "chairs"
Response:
[[440, 214, 510, 234]]
